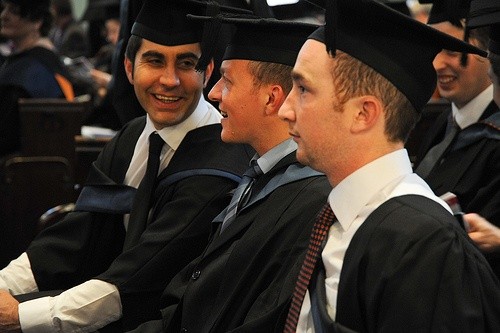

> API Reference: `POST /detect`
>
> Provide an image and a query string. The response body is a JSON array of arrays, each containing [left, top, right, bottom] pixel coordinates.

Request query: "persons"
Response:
[[206, 0, 499, 332], [1, 0, 500, 333], [120, 12, 333, 333], [1, 0, 258, 333]]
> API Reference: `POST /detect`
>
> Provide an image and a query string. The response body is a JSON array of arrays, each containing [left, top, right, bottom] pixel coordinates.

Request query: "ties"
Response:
[[219, 160, 263, 237], [413, 115, 460, 180], [284, 203, 338, 333], [122, 131, 167, 252]]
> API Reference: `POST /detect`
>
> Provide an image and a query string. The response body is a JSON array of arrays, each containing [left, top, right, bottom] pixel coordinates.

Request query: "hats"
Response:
[[5, 0, 53, 28], [130, 0, 254, 46], [467, 0, 500, 55], [305, 0, 488, 114], [104, 4, 120, 20], [186, 14, 322, 75], [418, 0, 470, 66]]
